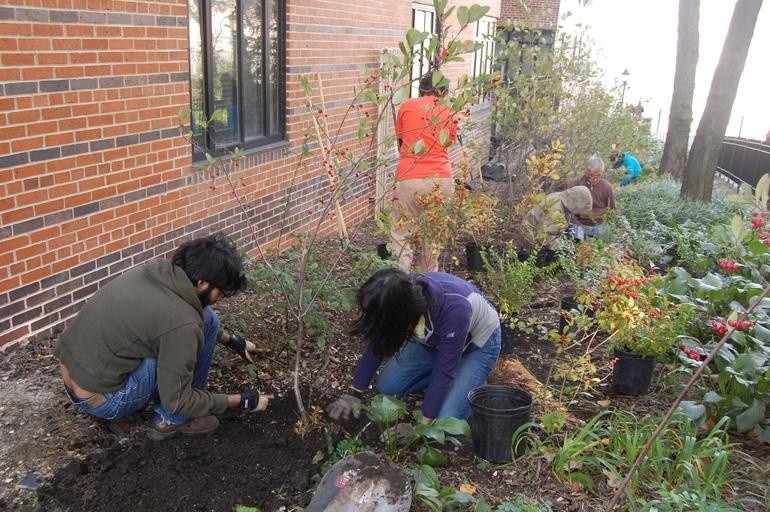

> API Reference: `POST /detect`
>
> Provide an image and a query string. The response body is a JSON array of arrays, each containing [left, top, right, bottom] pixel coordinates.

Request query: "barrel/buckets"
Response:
[[468, 384, 532, 464], [613, 346, 654, 398], [560, 298, 593, 333], [466, 241, 490, 272]]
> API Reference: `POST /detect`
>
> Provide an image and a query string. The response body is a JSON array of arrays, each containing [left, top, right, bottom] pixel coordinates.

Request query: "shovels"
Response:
[[304, 451, 418, 512]]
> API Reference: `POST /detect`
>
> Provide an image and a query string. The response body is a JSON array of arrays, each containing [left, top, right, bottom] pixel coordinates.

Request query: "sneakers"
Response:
[[147, 414, 220, 441]]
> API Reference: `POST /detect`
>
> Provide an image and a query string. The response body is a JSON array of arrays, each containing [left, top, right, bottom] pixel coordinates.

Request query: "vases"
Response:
[[467, 380, 539, 467]]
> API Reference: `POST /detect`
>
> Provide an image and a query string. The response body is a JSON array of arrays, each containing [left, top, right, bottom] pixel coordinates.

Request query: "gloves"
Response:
[[378, 421, 425, 446], [223, 333, 272, 364], [237, 389, 275, 414], [324, 387, 364, 421]]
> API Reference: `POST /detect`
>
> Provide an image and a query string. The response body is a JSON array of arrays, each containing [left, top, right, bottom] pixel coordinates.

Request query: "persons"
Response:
[[609, 150, 644, 187], [385, 72, 460, 273], [49, 232, 277, 437], [518, 183, 596, 274], [562, 153, 619, 246], [324, 265, 503, 429]]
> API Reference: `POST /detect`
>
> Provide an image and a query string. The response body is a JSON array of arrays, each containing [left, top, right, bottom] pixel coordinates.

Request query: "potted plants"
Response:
[[458, 190, 503, 272], [604, 285, 700, 398], [466, 231, 564, 356]]
